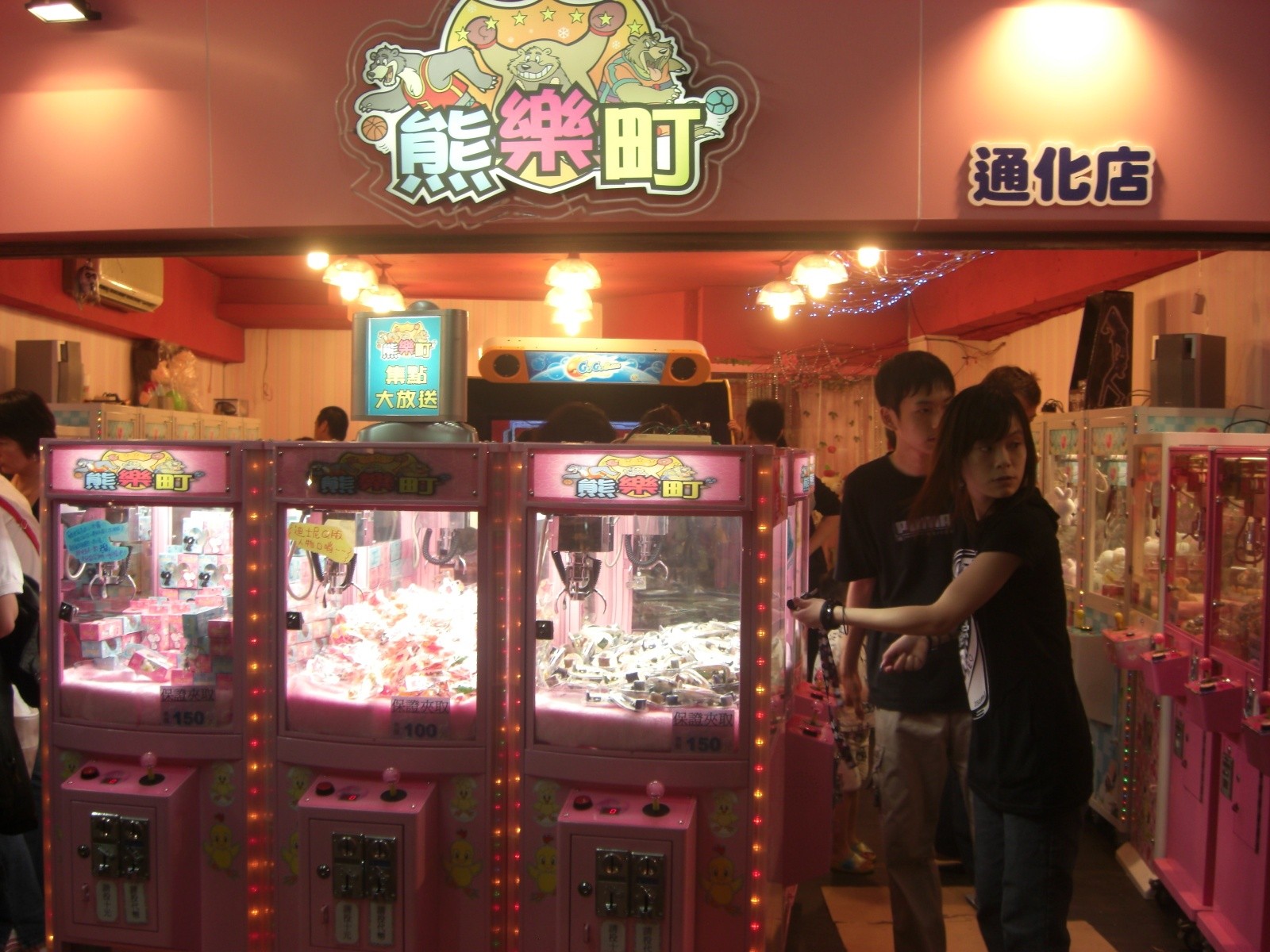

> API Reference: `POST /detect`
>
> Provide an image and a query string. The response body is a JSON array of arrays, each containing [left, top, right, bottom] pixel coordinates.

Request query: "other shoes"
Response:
[[938, 834, 976, 861]]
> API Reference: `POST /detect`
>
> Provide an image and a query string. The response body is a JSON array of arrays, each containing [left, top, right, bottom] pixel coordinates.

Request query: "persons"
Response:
[[0, 390, 55, 521], [313, 405, 350, 442], [807, 473, 844, 682], [983, 363, 1044, 422], [839, 351, 964, 952], [642, 405, 687, 437], [541, 402, 617, 442], [787, 382, 1089, 952], [0, 472, 42, 952], [745, 400, 788, 448]]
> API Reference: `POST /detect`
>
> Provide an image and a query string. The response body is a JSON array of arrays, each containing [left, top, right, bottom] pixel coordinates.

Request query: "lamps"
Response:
[[753, 246, 884, 322], [304, 247, 408, 324], [538, 251, 605, 338]]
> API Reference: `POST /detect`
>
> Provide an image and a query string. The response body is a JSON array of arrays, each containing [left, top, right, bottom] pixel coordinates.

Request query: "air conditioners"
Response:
[[59, 255, 166, 315]]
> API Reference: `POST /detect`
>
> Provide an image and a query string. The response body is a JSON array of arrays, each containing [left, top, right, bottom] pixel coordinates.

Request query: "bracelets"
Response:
[[819, 600, 839, 631]]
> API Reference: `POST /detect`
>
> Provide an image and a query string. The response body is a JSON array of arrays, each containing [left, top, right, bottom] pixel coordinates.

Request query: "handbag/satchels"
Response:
[[808, 628, 871, 792]]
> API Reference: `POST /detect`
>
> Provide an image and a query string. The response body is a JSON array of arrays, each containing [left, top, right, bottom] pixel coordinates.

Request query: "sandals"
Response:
[[854, 842, 878, 860], [831, 854, 877, 874]]
